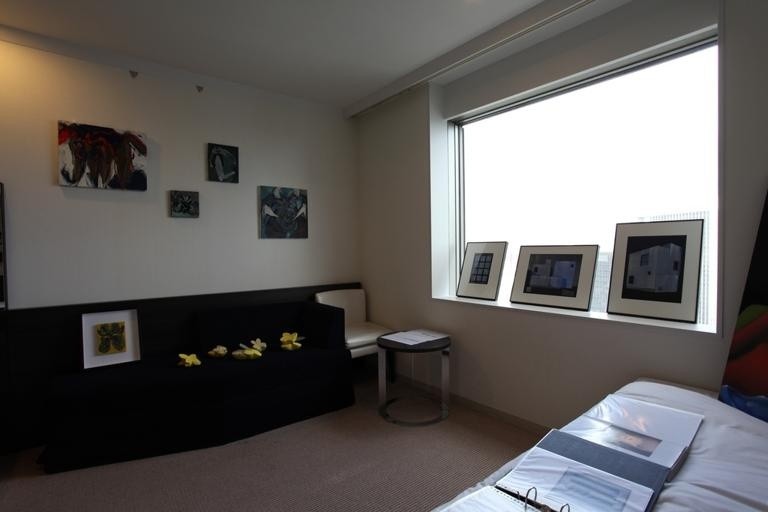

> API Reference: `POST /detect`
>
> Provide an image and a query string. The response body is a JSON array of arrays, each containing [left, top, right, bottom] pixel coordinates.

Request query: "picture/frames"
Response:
[[510, 245, 598, 311], [456, 241, 507, 300], [607, 219, 704, 323]]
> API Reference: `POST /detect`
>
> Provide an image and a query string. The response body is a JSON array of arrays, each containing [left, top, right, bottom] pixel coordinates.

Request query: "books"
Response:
[[559, 393, 706, 480], [435, 428, 671, 512]]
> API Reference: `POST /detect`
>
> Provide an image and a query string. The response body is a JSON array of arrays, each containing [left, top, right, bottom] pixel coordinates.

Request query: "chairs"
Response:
[[315, 289, 395, 404]]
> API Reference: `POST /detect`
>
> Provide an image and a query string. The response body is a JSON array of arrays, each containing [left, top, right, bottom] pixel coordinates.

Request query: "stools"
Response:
[[378, 330, 451, 427]]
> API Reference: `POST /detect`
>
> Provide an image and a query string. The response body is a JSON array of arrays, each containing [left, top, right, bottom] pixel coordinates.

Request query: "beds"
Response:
[[431, 377, 768, 512]]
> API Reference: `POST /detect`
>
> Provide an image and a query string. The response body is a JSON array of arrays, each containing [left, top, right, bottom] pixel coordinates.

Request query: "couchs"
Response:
[[3, 282, 361, 475]]
